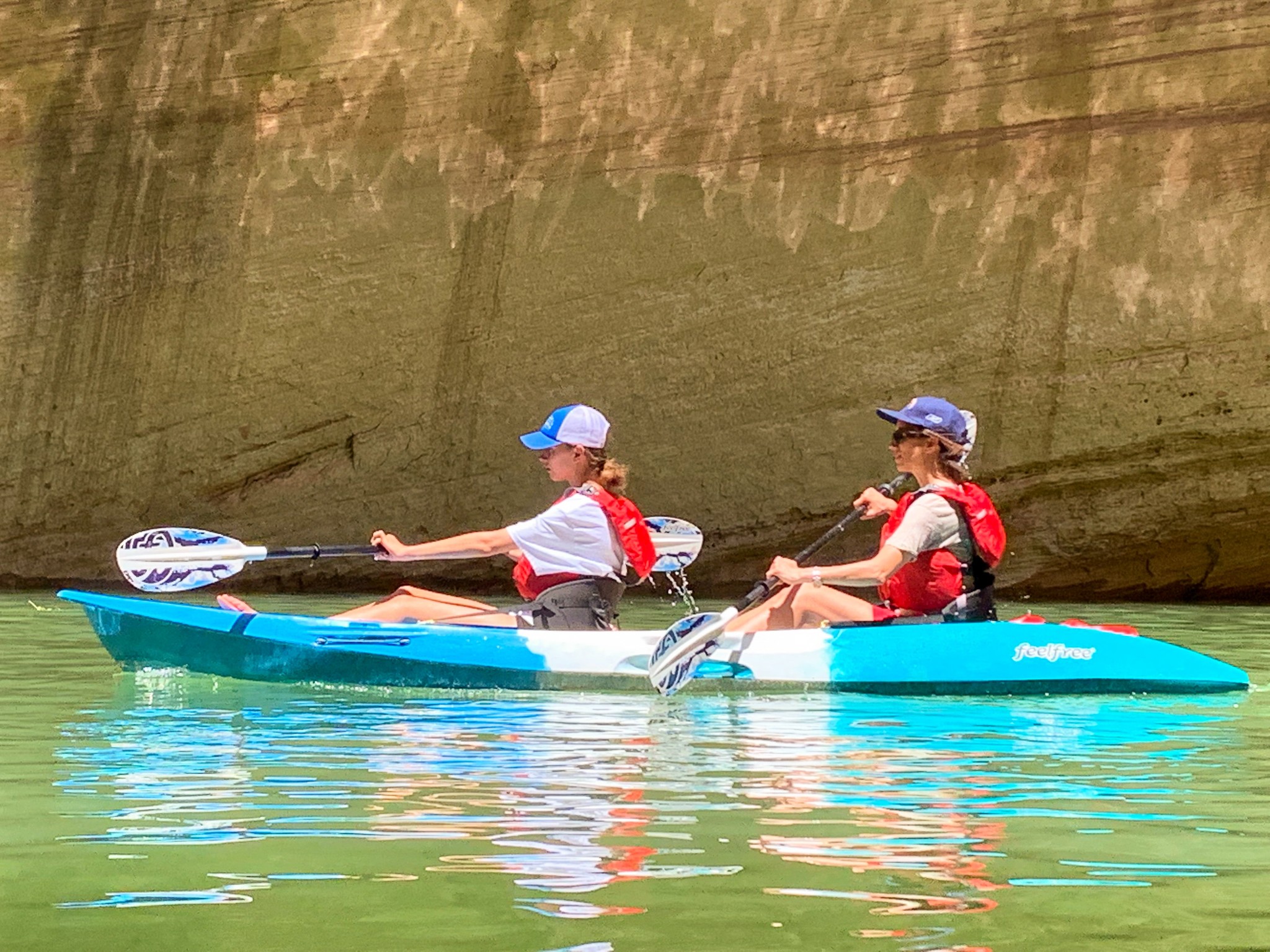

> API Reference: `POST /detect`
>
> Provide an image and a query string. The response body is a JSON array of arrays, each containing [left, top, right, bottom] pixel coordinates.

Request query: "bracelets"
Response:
[[811, 566, 822, 588]]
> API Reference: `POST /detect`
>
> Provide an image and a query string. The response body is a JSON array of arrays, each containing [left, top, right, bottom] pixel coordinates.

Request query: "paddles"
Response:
[[646, 406, 980, 700], [114, 515, 709, 594]]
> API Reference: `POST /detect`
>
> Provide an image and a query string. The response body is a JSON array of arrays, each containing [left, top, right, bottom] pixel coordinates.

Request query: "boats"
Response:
[[54, 585, 1252, 692]]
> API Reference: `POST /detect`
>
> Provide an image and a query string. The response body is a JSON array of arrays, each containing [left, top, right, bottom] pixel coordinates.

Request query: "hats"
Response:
[[877, 396, 966, 443], [519, 405, 610, 450]]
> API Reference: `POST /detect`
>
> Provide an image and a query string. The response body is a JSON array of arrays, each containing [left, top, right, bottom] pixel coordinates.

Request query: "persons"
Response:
[[216, 404, 625, 631], [724, 397, 984, 632]]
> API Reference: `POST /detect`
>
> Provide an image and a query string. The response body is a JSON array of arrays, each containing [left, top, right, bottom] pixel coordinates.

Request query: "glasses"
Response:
[[892, 427, 929, 442]]
